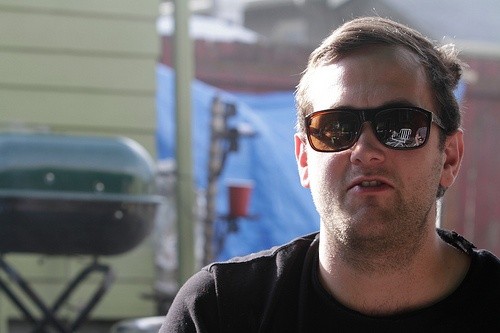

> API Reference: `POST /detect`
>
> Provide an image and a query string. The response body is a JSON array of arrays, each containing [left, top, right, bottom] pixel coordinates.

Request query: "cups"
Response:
[[228, 179, 252, 218]]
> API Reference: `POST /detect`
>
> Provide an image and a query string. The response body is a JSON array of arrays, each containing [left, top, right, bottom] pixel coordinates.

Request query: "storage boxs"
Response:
[[0, 132, 167, 260]]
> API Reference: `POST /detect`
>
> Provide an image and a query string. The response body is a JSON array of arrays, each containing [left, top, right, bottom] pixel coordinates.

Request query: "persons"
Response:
[[153, 14, 500, 333]]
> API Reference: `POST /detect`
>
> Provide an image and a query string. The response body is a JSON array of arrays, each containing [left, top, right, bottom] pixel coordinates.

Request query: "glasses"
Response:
[[303, 105, 448, 152]]
